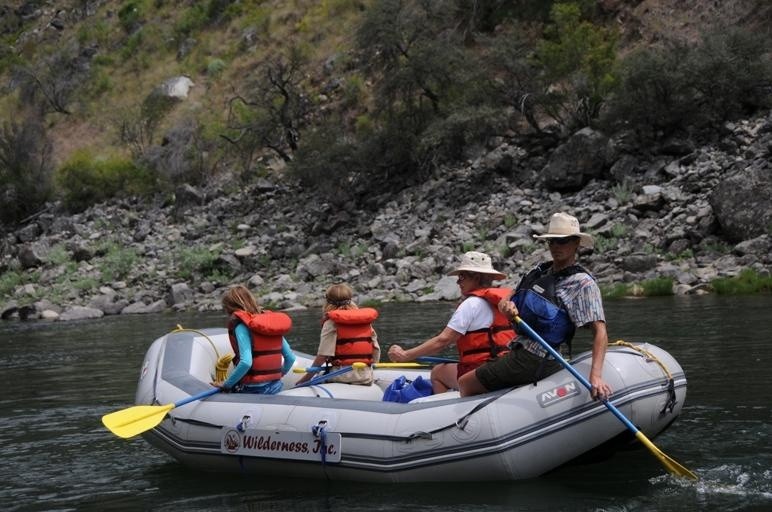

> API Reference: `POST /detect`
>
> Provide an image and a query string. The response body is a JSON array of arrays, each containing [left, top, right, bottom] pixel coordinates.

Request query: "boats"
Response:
[[135, 324, 687, 485]]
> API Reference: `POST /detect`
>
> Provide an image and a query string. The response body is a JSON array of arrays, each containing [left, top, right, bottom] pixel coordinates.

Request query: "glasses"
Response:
[[547, 236, 578, 244]]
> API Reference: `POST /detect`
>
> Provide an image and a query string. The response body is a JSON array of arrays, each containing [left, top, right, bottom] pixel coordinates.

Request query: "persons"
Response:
[[208, 284, 297, 396], [294, 284, 381, 389], [456, 211, 615, 404], [387, 251, 515, 396]]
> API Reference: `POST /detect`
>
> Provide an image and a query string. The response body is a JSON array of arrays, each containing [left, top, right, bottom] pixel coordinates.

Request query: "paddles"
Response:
[[293, 363, 430, 373], [513, 316, 698, 481], [102, 386, 222, 439]]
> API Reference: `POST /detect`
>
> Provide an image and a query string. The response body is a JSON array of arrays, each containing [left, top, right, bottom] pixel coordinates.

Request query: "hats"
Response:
[[532, 212, 594, 251], [447, 250, 506, 281]]
